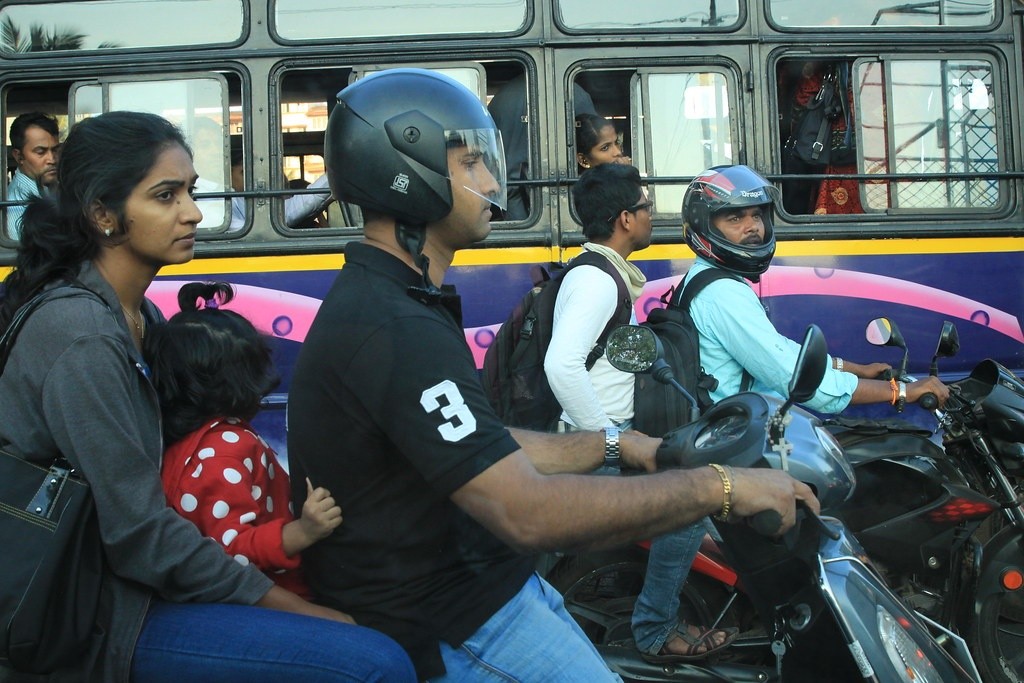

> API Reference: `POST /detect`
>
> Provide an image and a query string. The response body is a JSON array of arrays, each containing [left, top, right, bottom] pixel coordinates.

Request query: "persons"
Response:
[[286, 71, 820, 682], [184, 117, 333, 231], [156, 282, 341, 597], [542, 166, 738, 660], [7, 116, 61, 239], [670, 165, 949, 616], [0, 112, 418, 683], [777, 60, 892, 215], [487, 66, 596, 220], [574, 112, 633, 171]]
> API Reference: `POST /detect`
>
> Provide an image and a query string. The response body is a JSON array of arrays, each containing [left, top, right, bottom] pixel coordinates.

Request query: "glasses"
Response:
[[606, 201, 654, 223]]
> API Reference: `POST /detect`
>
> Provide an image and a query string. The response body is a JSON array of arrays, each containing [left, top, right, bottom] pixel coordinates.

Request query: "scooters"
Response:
[[540, 310, 1024, 683]]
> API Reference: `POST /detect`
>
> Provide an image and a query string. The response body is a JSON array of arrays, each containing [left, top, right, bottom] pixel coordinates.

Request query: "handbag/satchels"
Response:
[[0, 287, 121, 675], [783, 61, 843, 172]]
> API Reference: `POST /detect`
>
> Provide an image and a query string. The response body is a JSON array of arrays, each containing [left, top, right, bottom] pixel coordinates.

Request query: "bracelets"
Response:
[[709, 464, 736, 523], [833, 357, 844, 372], [890, 379, 906, 413]]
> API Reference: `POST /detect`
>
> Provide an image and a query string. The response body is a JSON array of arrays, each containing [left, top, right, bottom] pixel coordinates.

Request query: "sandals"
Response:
[[640, 622, 740, 664]]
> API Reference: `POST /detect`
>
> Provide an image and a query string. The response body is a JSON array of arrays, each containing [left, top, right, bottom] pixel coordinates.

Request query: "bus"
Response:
[[0, 0, 1024, 503]]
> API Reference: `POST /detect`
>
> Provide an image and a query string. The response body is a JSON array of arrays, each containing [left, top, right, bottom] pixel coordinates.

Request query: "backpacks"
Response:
[[481, 251, 632, 434], [631, 267, 770, 439]]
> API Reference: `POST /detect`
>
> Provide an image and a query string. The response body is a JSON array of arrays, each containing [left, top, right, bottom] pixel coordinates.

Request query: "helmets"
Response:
[[323, 67, 500, 224], [681, 164, 776, 274]]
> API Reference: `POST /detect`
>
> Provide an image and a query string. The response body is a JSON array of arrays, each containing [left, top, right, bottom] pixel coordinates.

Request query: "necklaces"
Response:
[[121, 303, 145, 345]]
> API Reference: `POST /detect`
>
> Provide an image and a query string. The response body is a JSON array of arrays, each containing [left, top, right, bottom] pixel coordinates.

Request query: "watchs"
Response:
[[599, 426, 624, 466]]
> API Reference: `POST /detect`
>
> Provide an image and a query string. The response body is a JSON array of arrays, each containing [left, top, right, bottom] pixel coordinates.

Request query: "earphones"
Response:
[[583, 160, 587, 164]]
